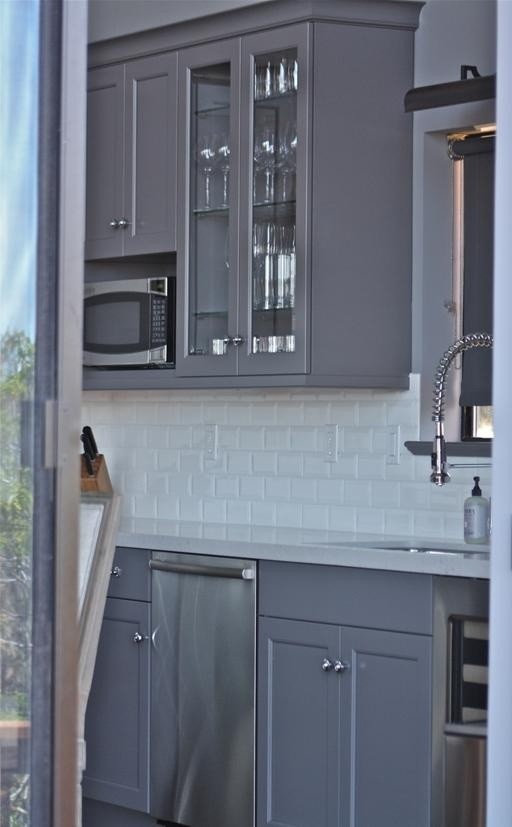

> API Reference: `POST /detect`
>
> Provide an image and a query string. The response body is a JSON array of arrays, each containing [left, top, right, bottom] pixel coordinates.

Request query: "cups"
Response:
[[210, 334, 294, 356], [223, 220, 295, 309], [253, 56, 298, 98]]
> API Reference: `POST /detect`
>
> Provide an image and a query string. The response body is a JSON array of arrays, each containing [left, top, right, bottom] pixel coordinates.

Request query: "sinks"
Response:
[[359, 545, 490, 562]]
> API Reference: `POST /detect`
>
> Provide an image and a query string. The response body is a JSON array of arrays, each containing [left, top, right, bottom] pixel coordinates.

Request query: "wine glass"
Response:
[[192, 119, 298, 211]]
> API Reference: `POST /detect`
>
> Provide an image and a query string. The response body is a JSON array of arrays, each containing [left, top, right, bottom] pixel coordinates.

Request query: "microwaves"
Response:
[[83, 276, 177, 369]]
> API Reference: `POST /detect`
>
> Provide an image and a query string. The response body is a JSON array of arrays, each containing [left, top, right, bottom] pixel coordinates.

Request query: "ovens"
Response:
[[143, 549, 258, 827]]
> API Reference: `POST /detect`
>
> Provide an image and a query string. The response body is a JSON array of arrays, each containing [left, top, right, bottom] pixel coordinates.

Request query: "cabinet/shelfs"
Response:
[[83, 546, 153, 826], [181, 1, 429, 392], [82, 20, 180, 393], [256, 558, 433, 827]]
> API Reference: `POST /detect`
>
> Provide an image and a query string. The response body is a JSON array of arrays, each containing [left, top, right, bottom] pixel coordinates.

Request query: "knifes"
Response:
[[79, 425, 101, 479]]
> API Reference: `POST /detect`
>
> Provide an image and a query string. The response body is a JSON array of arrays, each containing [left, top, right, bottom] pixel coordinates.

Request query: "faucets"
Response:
[[429, 330, 493, 488]]
